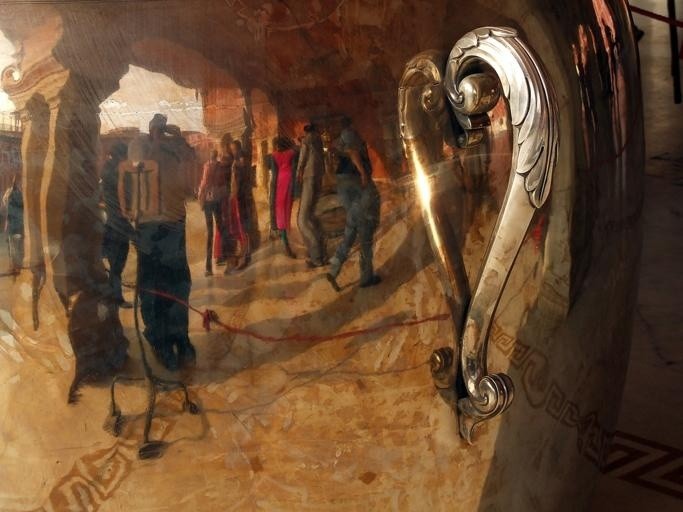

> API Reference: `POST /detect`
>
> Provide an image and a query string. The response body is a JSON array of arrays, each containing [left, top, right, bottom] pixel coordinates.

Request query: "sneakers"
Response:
[[325, 271, 382, 292]]
